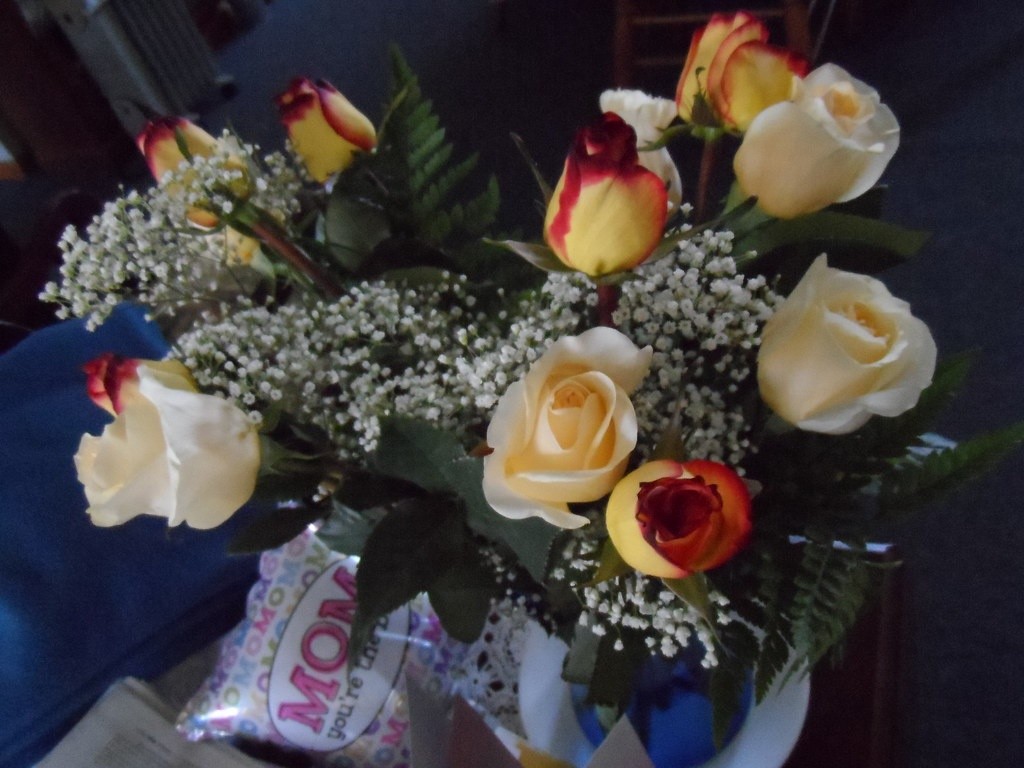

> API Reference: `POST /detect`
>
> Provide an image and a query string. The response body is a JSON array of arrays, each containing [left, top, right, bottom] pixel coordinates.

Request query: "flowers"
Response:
[[35, 11, 1024, 751]]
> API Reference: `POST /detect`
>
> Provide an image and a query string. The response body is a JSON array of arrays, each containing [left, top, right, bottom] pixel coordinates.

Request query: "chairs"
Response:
[[604, 1, 836, 225], [0, 293, 270, 766]]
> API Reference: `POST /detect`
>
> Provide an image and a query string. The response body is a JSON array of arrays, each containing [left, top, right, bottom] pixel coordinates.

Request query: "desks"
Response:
[[784, 533, 920, 768]]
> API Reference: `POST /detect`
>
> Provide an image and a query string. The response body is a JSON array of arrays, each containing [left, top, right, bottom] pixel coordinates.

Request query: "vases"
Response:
[[568, 635, 753, 768]]
